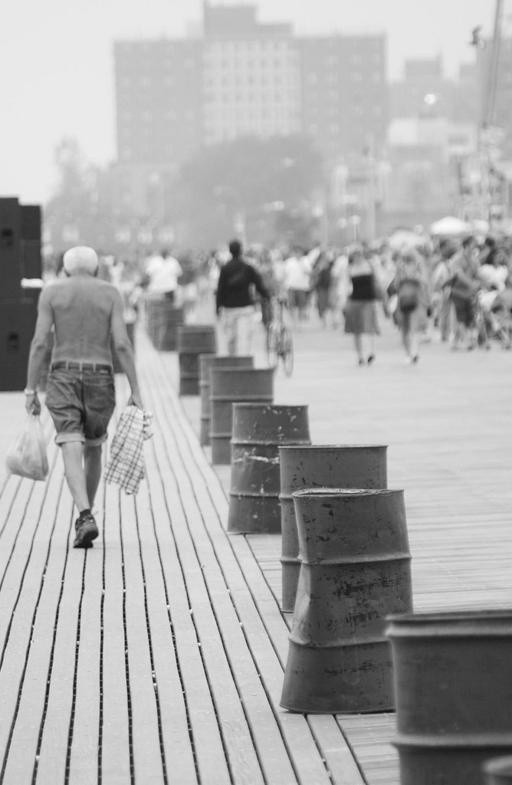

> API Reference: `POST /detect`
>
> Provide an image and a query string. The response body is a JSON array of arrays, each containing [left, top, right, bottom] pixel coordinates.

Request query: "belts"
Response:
[[50, 361, 113, 374]]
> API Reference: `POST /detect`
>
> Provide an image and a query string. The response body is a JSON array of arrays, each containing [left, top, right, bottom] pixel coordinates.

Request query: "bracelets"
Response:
[[25, 389, 36, 396]]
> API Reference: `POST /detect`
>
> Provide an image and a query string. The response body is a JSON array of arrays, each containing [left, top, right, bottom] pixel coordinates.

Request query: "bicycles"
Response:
[[268, 295, 296, 378]]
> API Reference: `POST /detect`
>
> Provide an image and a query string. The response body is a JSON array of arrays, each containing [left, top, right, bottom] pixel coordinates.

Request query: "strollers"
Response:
[[470, 288, 511, 353]]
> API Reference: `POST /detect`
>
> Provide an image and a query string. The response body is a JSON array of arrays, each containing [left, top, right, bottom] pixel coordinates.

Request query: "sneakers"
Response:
[[410, 354, 420, 364], [73, 513, 99, 548], [358, 353, 377, 367], [440, 333, 475, 351]]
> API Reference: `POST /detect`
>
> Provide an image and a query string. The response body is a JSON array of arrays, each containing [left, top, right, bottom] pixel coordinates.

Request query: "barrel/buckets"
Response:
[[176, 325, 218, 398], [197, 354, 253, 448], [382, 608, 511, 785], [157, 308, 184, 352], [274, 443, 389, 617], [223, 401, 310, 537], [277, 486, 415, 716], [209, 366, 274, 466]]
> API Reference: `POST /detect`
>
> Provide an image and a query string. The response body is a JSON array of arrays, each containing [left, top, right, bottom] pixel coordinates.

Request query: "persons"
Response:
[[25, 247, 144, 548]]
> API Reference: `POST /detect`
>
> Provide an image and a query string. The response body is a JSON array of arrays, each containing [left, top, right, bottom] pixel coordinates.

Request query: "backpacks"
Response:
[[398, 268, 419, 311]]
